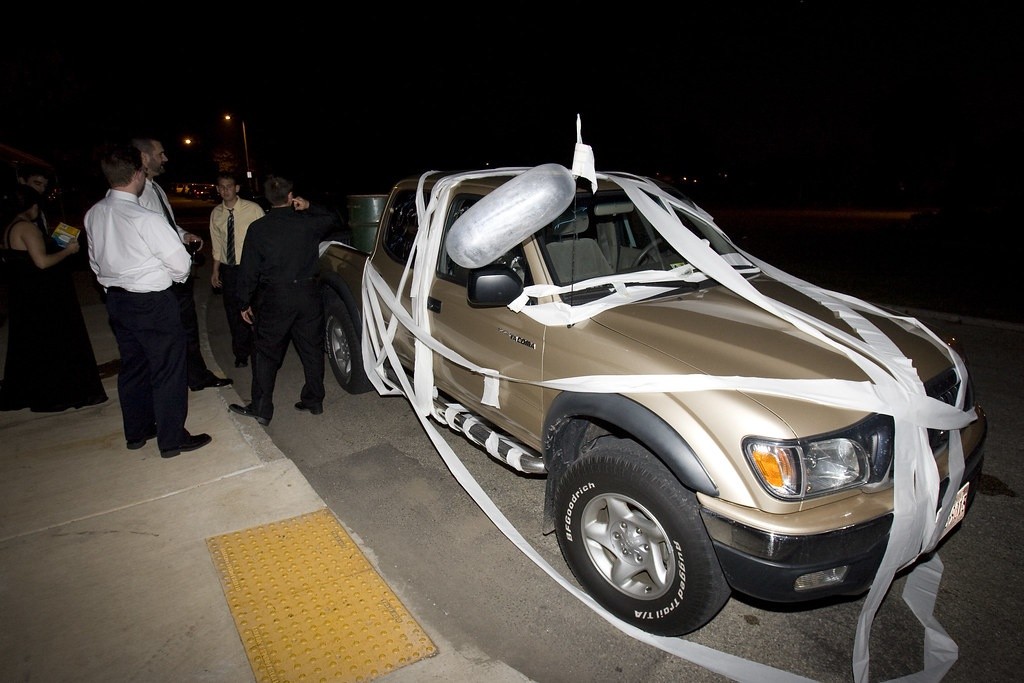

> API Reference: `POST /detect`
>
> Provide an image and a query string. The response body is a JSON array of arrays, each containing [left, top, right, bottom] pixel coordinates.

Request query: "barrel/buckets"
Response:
[[347, 195, 388, 254]]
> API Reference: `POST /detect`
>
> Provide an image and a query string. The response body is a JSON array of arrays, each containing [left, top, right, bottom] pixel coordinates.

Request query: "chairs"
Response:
[[546, 211, 614, 285]]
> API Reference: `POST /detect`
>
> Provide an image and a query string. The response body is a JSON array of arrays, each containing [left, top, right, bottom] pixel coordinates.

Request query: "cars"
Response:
[[171, 181, 223, 202]]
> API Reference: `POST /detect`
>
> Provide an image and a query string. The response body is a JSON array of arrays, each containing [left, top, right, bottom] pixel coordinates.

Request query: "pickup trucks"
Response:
[[303, 170, 994, 637]]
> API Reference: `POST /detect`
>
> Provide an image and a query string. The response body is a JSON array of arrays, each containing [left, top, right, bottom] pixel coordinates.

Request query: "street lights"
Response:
[[223, 114, 252, 175]]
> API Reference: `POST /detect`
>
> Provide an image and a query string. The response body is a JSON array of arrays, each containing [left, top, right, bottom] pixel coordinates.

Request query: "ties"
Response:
[[36, 209, 48, 241], [227, 208, 236, 267], [152, 182, 182, 242]]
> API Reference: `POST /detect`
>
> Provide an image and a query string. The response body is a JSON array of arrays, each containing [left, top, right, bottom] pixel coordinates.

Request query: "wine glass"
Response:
[[190, 237, 201, 264]]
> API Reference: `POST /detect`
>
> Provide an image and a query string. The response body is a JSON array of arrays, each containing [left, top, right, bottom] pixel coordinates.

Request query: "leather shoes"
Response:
[[229, 404, 271, 426], [127, 429, 157, 449], [191, 374, 234, 391], [295, 402, 323, 415], [161, 433, 212, 458]]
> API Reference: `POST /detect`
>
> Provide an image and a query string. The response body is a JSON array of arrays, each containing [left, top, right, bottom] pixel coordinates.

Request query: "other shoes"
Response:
[[235, 357, 248, 368]]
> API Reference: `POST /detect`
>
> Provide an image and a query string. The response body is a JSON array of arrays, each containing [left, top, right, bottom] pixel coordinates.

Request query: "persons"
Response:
[[230, 178, 337, 426], [129, 138, 234, 392], [208, 174, 266, 368], [83, 143, 212, 459], [0, 163, 108, 413]]
